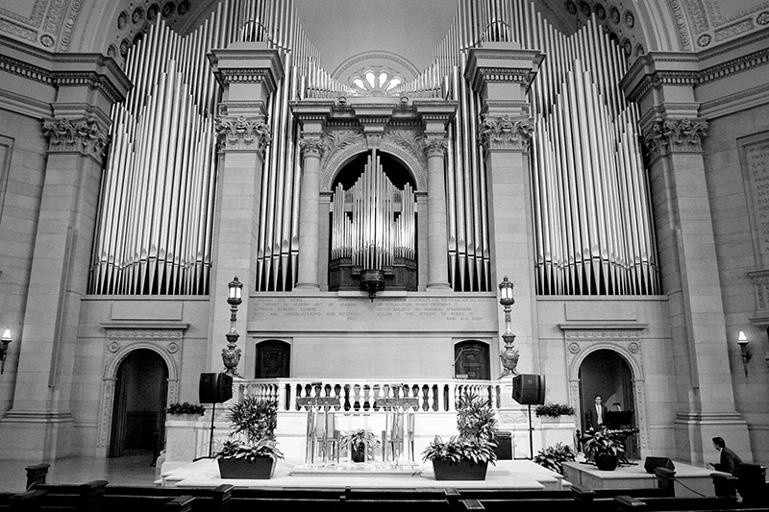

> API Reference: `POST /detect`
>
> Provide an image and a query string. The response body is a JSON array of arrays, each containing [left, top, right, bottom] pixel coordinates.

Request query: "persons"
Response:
[[585, 394, 609, 435], [704, 437, 746, 499], [148, 428, 164, 467]]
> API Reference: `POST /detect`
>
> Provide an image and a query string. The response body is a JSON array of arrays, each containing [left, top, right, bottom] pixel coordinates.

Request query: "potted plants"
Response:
[[423, 434, 499, 483], [584, 430, 626, 471], [165, 401, 207, 422], [535, 402, 576, 423], [211, 437, 284, 480]]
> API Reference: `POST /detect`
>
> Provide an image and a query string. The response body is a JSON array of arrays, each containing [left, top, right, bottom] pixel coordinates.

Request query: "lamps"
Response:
[[221, 274, 245, 378], [1, 329, 12, 375], [738, 329, 752, 381], [497, 275, 520, 381]]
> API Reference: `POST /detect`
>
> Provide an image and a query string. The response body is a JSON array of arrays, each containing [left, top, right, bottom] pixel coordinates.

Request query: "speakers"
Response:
[[511, 374, 546, 405], [199, 371, 233, 403]]
